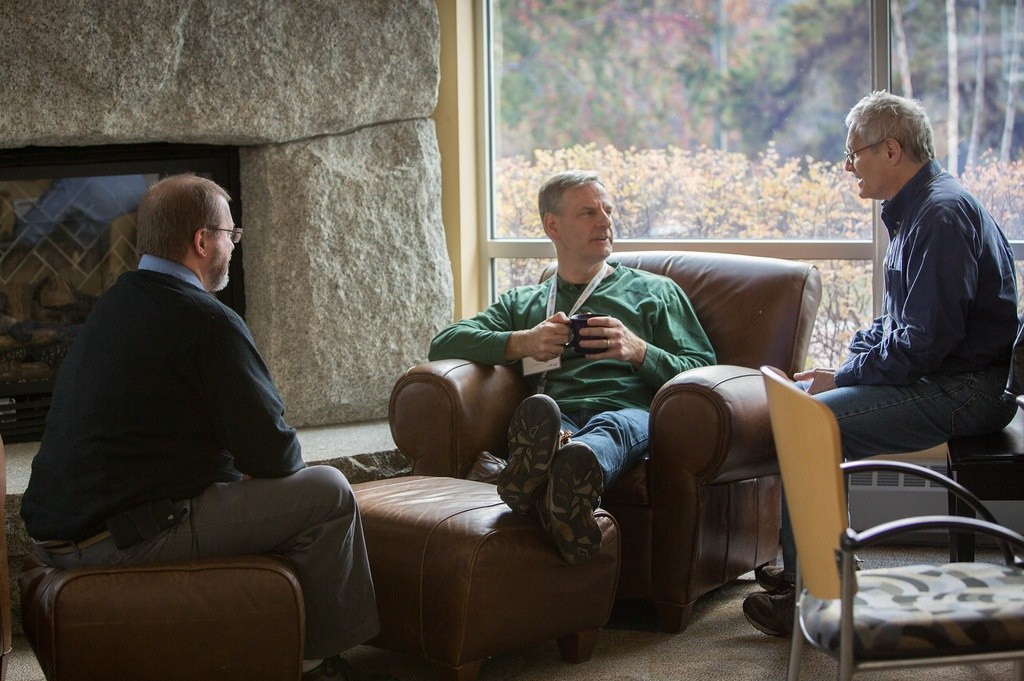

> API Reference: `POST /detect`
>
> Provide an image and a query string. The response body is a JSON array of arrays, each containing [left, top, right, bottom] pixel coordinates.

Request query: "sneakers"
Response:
[[497, 393, 561, 514], [546, 444, 603, 564], [758, 565, 796, 591], [743, 584, 796, 637]]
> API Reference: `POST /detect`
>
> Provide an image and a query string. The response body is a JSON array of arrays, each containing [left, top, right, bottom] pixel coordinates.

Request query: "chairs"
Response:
[[759, 364, 1023, 680]]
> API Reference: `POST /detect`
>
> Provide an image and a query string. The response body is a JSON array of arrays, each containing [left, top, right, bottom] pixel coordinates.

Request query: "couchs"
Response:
[[388, 249, 822, 635]]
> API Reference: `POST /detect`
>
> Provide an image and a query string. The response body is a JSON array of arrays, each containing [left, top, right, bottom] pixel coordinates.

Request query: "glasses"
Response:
[[204, 227, 244, 243], [844, 138, 887, 163]]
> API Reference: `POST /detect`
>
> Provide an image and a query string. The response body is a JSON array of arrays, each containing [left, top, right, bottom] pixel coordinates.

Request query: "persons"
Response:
[[428, 171, 717, 566], [20, 172, 381, 681], [742, 92, 1017, 638]]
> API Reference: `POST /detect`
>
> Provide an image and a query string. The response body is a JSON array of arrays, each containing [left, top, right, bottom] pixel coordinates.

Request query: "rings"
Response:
[[607, 339, 610, 349]]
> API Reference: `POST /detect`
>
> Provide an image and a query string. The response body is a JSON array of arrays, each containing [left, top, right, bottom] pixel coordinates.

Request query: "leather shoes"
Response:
[[301, 655, 396, 681]]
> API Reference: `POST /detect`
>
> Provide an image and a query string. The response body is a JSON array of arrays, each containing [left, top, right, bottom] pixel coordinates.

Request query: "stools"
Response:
[[16, 556, 303, 681], [326, 476, 620, 680], [947, 403, 1023, 562]]
